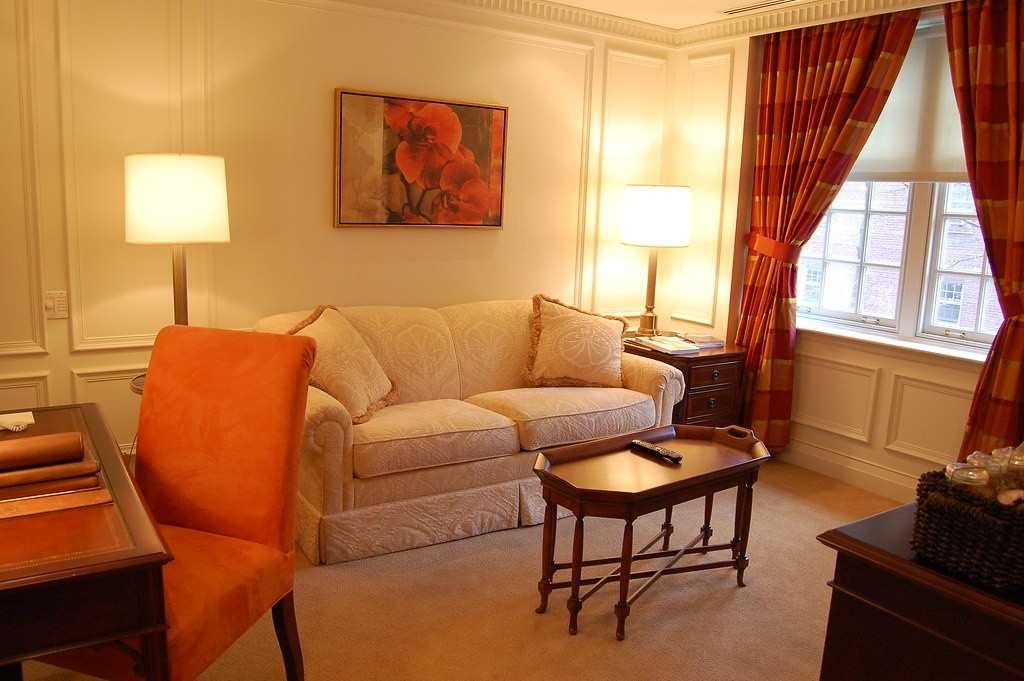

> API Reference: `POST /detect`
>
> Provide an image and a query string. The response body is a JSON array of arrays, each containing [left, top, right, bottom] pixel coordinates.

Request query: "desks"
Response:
[[0, 401, 176, 681], [127, 373, 146, 464], [815, 499, 1024, 681]]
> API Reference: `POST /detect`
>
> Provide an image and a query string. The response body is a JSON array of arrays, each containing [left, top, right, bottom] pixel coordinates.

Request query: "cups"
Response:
[[944, 442, 1024, 501]]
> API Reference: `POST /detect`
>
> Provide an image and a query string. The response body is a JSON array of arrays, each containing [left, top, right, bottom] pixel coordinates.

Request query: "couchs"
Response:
[[255, 299, 685, 566]]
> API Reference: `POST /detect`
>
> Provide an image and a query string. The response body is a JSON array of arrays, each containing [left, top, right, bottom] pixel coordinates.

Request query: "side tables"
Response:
[[622, 338, 747, 427]]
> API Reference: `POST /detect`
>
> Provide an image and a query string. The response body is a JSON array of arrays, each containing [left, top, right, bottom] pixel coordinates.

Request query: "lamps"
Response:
[[619, 184, 692, 338], [123, 153, 231, 325]]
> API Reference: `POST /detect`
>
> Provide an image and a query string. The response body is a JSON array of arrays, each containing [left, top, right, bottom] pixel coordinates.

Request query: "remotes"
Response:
[[632, 440, 683, 463]]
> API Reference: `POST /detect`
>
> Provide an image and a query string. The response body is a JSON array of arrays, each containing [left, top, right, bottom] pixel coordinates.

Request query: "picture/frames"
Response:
[[332, 88, 509, 230]]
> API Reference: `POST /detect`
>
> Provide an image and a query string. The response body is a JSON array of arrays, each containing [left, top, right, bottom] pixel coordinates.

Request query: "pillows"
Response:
[[521, 293, 630, 388], [286, 305, 400, 424]]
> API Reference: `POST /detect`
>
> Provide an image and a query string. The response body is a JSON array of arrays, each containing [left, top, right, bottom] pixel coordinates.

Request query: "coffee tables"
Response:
[[533, 424, 770, 640]]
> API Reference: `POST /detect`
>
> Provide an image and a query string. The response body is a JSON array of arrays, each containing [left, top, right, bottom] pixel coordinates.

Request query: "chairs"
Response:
[[30, 325, 318, 681]]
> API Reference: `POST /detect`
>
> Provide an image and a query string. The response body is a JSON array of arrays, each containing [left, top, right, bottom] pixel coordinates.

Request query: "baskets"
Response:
[[909, 446, 1024, 605]]
